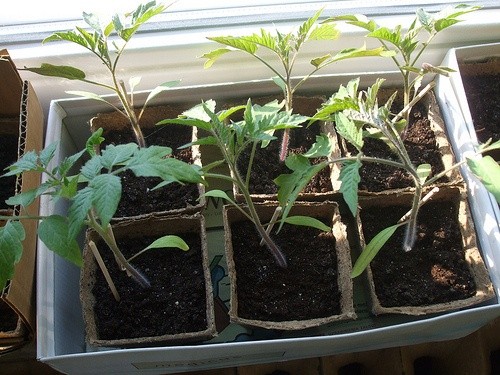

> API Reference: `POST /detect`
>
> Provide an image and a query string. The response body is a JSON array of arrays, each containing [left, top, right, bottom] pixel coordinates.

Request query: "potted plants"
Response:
[[317, 3, 468, 188], [133, 88, 356, 326], [0, 130, 221, 339], [196, 10, 340, 195], [19, 1, 208, 218], [265, 73, 497, 315]]
